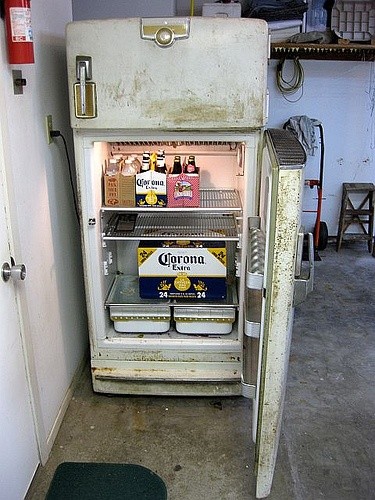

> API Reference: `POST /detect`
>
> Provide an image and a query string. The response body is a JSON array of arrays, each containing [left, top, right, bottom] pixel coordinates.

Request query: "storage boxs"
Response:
[[136, 228, 227, 301]]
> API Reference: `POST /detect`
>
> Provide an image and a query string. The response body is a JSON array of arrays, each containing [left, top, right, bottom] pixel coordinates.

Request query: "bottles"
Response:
[[106, 148, 198, 176]]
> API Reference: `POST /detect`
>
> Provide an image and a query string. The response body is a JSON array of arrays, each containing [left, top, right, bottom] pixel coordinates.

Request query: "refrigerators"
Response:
[[60, 15, 316, 498]]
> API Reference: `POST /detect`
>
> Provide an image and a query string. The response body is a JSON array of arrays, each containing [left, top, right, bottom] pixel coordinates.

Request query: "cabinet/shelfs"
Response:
[[271, 43, 375, 62]]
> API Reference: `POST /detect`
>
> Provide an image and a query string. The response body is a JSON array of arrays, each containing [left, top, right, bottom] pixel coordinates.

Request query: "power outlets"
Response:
[[45, 114, 54, 145]]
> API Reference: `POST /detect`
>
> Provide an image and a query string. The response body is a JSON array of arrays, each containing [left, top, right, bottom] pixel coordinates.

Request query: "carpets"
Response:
[[45, 462, 167, 499]]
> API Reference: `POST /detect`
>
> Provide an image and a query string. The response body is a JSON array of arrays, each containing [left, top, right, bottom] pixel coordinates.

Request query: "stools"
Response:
[[336, 183, 375, 257]]
